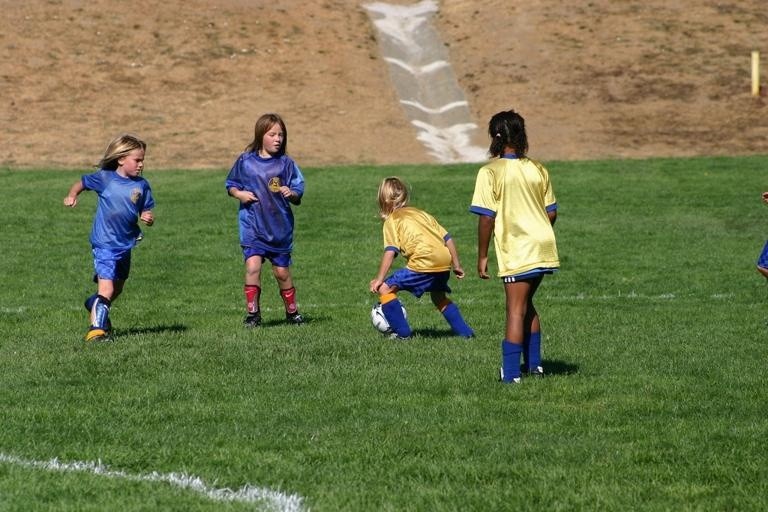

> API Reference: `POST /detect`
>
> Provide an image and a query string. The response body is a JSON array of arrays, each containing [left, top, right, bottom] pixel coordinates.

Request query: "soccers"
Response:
[[371, 302, 407, 335]]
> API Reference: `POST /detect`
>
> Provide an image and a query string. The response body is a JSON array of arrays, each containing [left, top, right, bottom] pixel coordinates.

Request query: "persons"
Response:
[[469, 110, 558, 387], [223, 113, 306, 329], [364, 175, 476, 340], [752, 189, 768, 279], [61, 133, 155, 343]]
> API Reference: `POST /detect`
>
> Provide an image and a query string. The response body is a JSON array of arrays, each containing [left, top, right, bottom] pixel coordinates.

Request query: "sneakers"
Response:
[[286, 310, 304, 324], [242, 311, 261, 327], [85, 294, 111, 335], [85, 325, 105, 342]]
